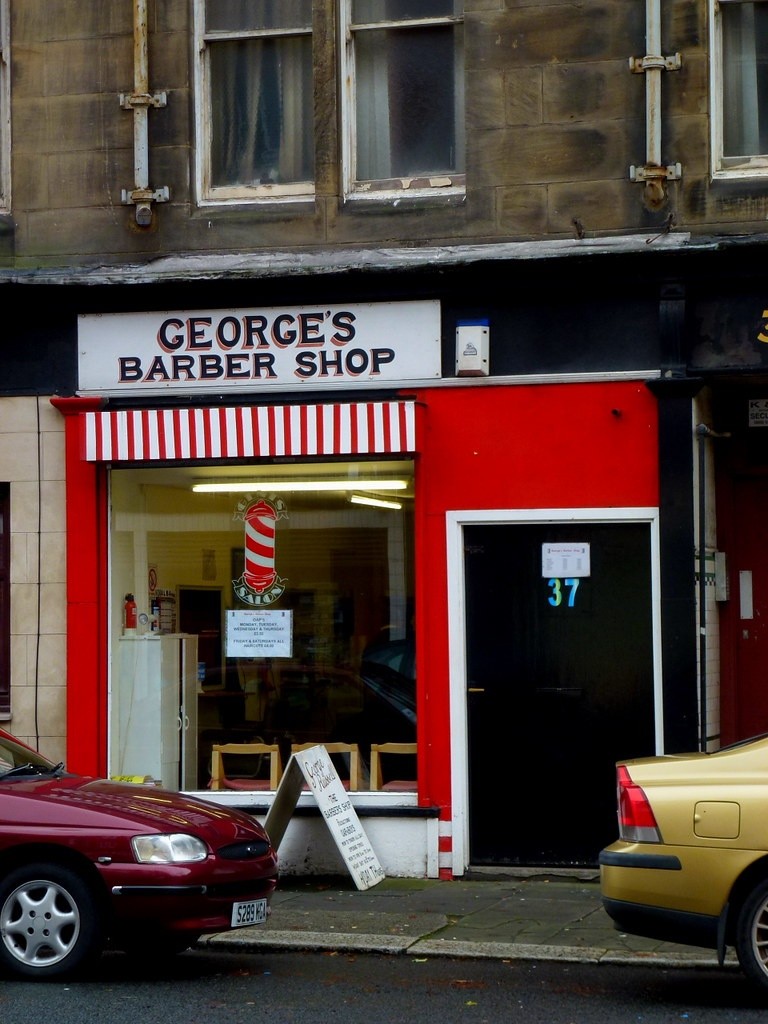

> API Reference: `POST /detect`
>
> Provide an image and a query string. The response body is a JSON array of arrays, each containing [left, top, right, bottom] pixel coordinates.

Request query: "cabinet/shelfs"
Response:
[[118, 632, 202, 793]]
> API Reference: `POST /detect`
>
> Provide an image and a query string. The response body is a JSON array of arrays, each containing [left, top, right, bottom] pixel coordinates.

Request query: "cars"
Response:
[[195, 628, 493, 788], [0, 731, 279, 983], [599, 734, 767, 982]]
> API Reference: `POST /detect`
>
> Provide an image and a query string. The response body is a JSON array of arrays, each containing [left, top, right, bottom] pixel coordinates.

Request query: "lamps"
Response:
[[186, 471, 412, 498], [342, 490, 404, 511], [137, 612, 156, 634]]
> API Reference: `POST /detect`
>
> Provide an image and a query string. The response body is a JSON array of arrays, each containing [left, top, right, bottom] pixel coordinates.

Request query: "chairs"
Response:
[[371, 740, 418, 793], [290, 739, 368, 792], [209, 741, 282, 792]]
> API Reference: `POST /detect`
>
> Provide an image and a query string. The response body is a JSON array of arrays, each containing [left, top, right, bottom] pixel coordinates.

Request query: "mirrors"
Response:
[[176, 581, 227, 689]]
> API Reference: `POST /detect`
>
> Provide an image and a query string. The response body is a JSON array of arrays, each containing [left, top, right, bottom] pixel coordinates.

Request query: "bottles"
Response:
[[153, 607, 159, 631], [125, 593, 137, 629]]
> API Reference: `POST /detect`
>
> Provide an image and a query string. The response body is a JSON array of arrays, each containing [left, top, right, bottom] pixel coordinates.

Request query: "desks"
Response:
[[198, 690, 255, 697]]
